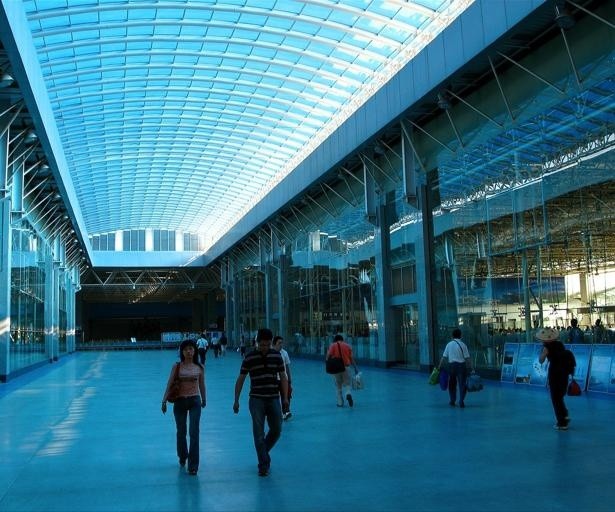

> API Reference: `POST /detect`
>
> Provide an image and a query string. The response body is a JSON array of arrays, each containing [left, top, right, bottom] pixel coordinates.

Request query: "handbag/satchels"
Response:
[[565, 350, 576, 375], [325, 357, 345, 374], [352, 373, 363, 390], [428, 367, 439, 384], [465, 375, 482, 392], [439, 370, 448, 391], [567, 381, 581, 397], [167, 382, 179, 402]]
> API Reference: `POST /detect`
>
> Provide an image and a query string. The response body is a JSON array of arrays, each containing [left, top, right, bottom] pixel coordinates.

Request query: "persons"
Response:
[[177, 331, 227, 369], [237, 333, 259, 357], [536, 325, 574, 430], [161, 339, 205, 475], [270, 335, 293, 422], [437, 329, 476, 408], [488, 319, 613, 354], [233, 328, 290, 477], [326, 334, 358, 407]]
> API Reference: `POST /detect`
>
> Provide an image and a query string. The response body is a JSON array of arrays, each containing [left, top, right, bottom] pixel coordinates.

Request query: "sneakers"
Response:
[[180, 457, 197, 475], [259, 462, 271, 476], [337, 393, 353, 407], [449, 401, 465, 408], [282, 412, 291, 420], [553, 416, 569, 430]]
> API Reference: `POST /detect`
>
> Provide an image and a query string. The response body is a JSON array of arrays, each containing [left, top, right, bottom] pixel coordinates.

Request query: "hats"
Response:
[[535, 326, 559, 341]]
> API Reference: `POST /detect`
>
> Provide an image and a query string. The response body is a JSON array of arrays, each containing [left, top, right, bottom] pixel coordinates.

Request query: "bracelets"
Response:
[[202, 400, 207, 402], [162, 401, 166, 404]]
